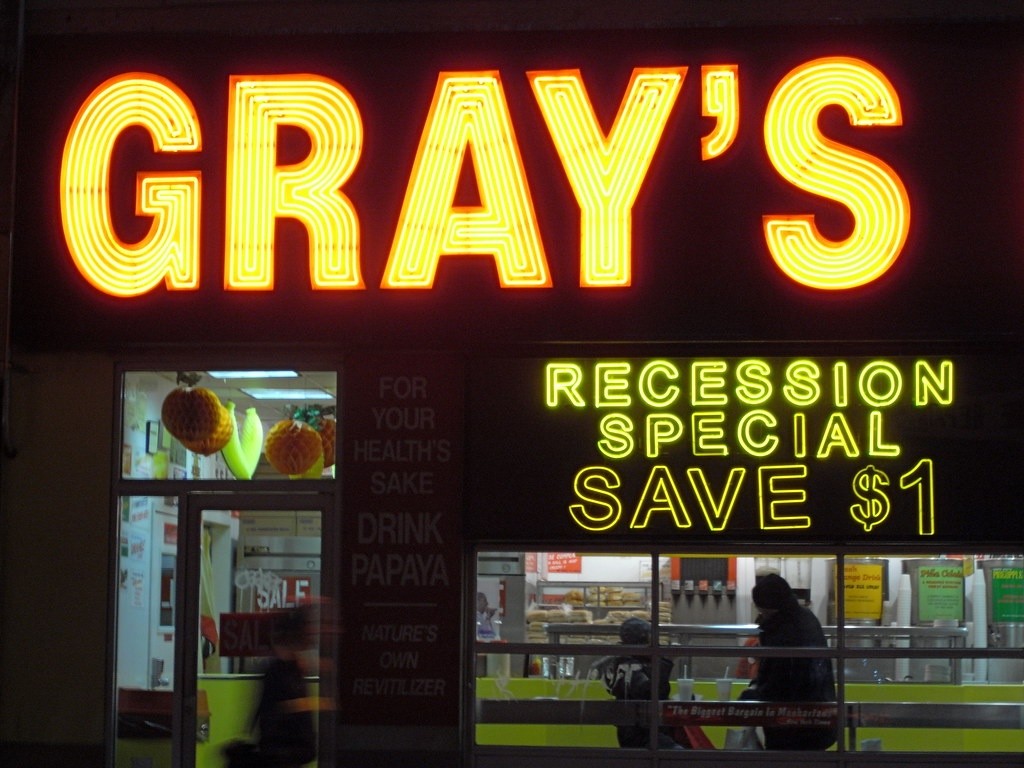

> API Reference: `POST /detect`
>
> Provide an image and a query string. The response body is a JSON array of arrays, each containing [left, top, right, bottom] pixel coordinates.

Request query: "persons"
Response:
[[735, 614, 761, 679], [738, 574, 838, 751], [477, 592, 495, 636], [602, 618, 675, 749]]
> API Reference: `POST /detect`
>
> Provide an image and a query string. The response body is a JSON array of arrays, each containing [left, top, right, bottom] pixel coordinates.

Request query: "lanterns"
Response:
[[161, 386, 233, 456], [265, 417, 337, 474]]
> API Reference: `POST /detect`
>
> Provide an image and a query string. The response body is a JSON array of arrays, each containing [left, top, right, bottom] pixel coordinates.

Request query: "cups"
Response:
[[559, 657, 574, 679], [717, 680, 733, 701], [677, 678, 695, 701], [881, 574, 911, 682], [967, 570, 987, 681]]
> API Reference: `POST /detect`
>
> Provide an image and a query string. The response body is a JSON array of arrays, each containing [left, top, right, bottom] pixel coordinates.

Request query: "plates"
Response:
[[923, 664, 951, 682], [934, 618, 959, 628]]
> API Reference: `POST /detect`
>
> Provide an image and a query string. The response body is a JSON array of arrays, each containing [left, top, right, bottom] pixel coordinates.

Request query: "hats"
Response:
[[620, 617, 652, 645]]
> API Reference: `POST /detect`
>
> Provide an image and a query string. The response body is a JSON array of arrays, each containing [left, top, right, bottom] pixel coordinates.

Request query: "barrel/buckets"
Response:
[[832, 619, 876, 647]]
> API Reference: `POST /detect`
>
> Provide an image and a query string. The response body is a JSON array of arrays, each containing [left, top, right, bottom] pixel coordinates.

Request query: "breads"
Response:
[[526, 584, 673, 647]]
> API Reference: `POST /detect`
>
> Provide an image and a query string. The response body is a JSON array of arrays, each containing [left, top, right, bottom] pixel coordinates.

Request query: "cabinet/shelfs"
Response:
[[535, 579, 665, 622]]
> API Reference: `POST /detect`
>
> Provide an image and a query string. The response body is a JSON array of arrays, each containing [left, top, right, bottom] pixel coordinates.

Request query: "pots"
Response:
[[989, 622, 1024, 648]]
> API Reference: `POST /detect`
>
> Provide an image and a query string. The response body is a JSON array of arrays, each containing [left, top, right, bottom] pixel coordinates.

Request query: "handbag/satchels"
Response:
[[674, 726, 715, 750], [723, 726, 766, 751]]
